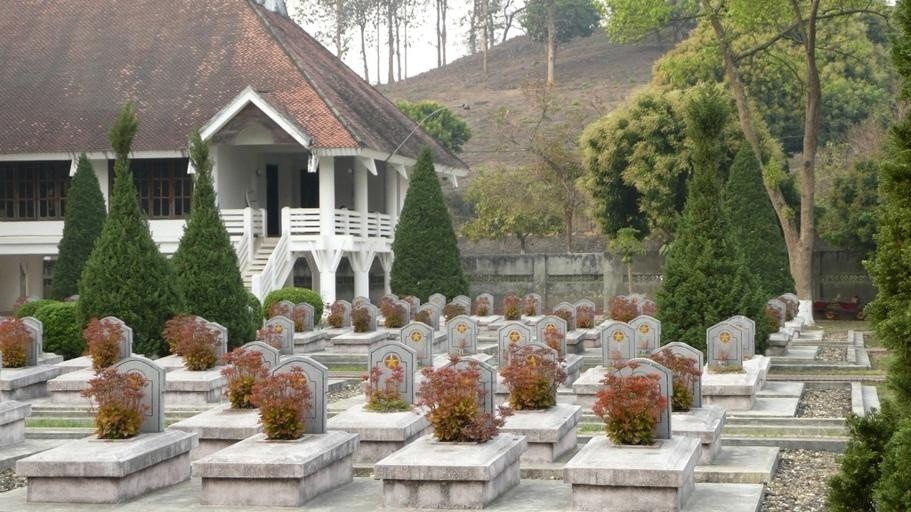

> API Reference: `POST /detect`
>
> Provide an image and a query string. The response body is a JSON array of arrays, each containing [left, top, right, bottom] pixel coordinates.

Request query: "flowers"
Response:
[[0, 317, 126, 369]]
